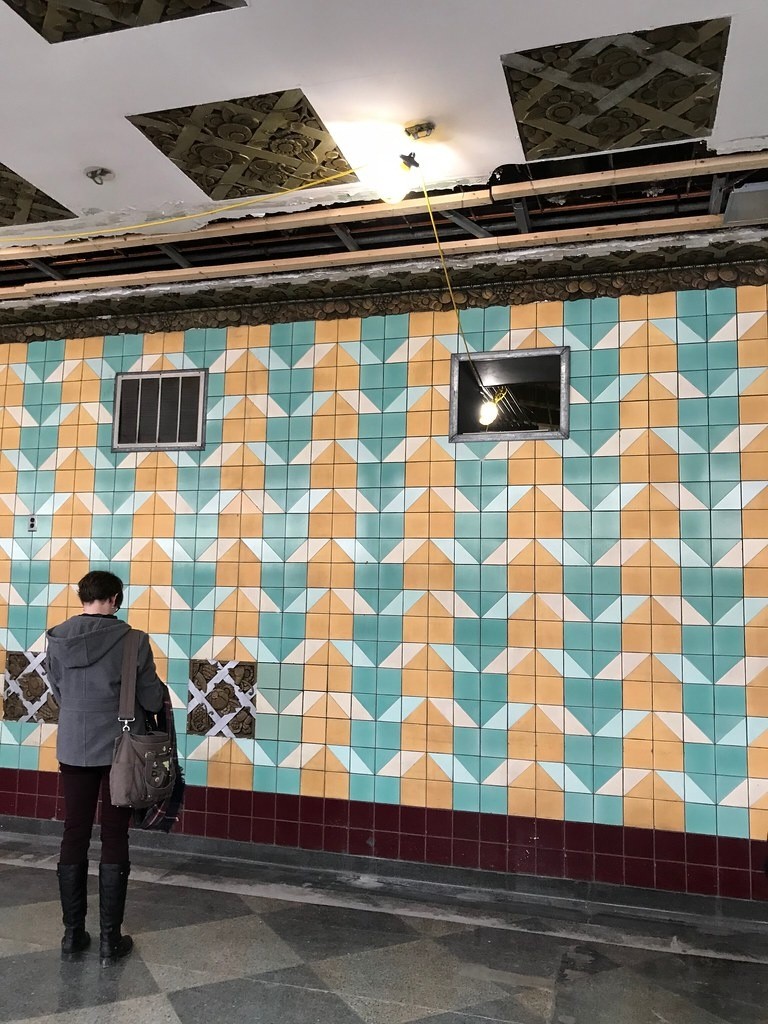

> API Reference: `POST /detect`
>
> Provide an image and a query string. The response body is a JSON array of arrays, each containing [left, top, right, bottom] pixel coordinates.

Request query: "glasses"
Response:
[[115, 606, 120, 613]]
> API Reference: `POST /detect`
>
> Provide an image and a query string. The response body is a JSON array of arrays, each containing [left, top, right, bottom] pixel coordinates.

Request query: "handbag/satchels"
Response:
[[111, 624, 179, 808]]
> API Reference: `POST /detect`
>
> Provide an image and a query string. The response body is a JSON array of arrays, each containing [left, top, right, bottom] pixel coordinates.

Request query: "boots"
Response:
[[55, 861, 92, 959], [98, 862, 134, 958]]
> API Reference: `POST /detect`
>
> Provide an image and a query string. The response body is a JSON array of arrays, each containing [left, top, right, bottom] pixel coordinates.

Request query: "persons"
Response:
[[46, 571, 164, 968]]
